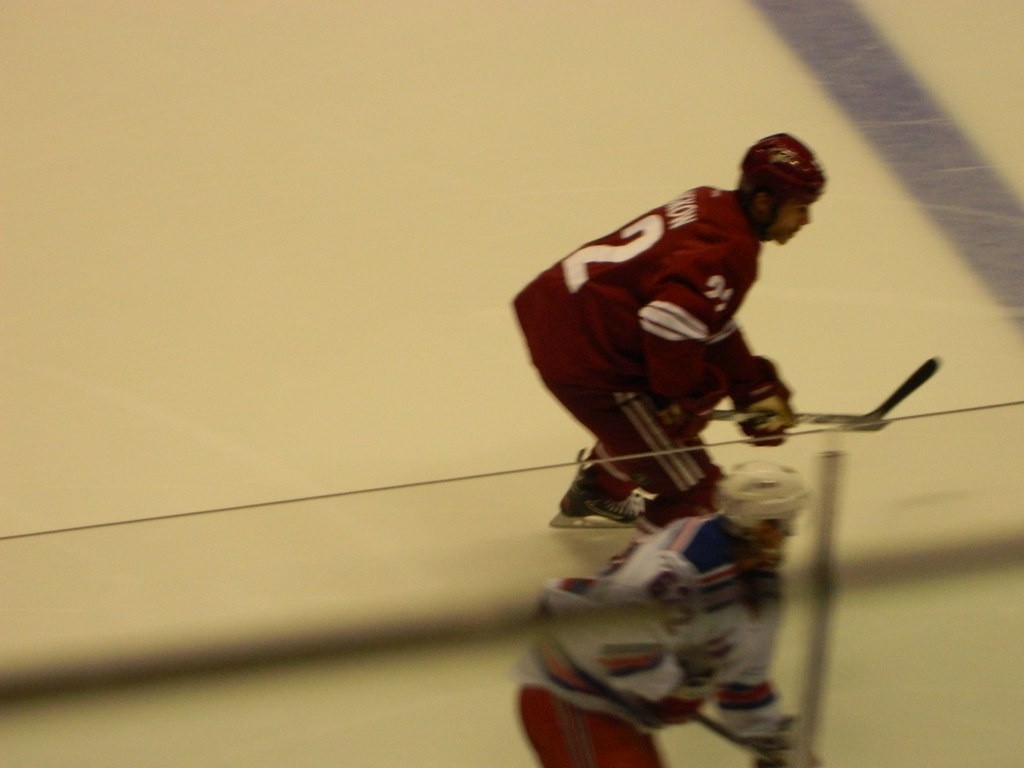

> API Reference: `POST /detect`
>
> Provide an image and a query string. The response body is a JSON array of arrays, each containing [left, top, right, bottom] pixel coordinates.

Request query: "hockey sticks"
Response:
[[689, 357, 942, 436], [688, 709, 794, 768]]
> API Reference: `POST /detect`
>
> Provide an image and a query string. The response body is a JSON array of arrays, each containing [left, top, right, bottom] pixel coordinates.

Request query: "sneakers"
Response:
[[634, 503, 673, 536], [548, 466, 649, 528]]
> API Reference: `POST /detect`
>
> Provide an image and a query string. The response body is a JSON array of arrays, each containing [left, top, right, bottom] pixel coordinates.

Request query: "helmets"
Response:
[[714, 461, 811, 530], [741, 133, 824, 205]]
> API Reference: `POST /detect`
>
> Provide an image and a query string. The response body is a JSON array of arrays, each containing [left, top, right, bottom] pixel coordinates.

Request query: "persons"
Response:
[[512, 133, 828, 557], [518, 458, 813, 768]]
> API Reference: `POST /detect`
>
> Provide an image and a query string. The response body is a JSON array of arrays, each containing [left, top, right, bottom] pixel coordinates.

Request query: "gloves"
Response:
[[652, 399, 712, 449], [734, 363, 794, 445]]
